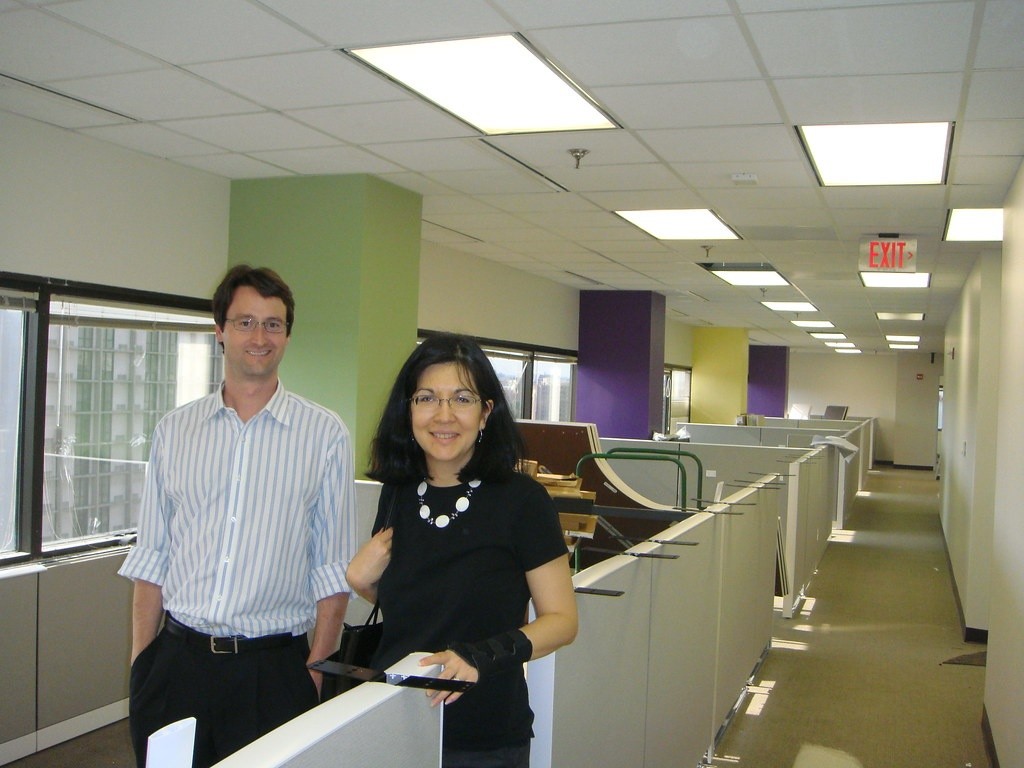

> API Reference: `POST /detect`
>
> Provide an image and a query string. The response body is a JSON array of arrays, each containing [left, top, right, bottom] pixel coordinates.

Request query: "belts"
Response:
[[164, 612, 309, 655]]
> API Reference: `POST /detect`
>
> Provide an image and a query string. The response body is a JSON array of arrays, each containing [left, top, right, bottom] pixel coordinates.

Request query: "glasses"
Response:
[[407, 389, 482, 409], [224, 315, 288, 333]]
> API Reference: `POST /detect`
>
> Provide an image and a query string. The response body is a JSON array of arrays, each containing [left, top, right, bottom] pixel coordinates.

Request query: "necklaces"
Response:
[[417, 477, 481, 528]]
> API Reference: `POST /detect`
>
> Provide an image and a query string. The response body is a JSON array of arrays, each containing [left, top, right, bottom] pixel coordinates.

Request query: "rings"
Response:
[[451, 677, 459, 680]]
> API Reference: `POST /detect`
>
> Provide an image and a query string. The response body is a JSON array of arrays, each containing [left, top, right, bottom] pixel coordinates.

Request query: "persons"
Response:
[[346, 334, 580, 768], [118, 264, 359, 768]]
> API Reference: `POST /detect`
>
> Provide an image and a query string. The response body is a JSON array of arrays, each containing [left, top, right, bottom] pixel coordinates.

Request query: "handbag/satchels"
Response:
[[340, 624, 384, 694]]
